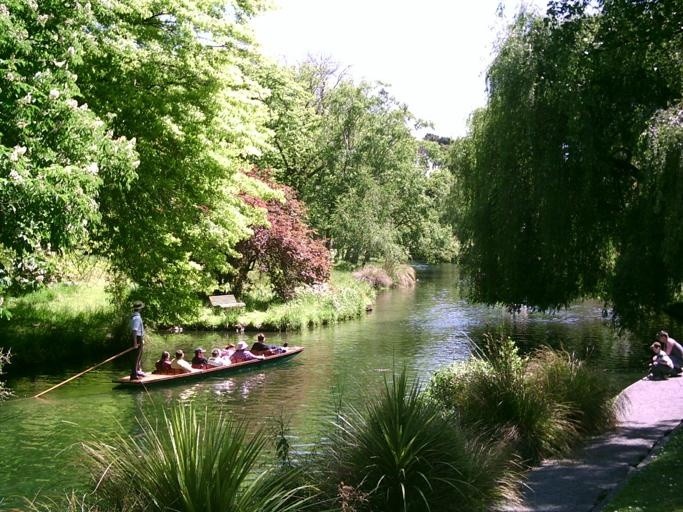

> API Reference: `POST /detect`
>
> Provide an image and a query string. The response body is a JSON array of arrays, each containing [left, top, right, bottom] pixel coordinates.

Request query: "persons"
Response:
[[250, 333, 274, 355], [657, 330, 683, 377], [128, 301, 146, 380], [192, 341, 266, 369], [155, 350, 172, 372], [170, 349, 194, 372], [648, 341, 675, 380]]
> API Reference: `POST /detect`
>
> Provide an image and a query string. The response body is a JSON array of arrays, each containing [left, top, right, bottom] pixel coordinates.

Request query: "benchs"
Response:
[[208, 294, 247, 314]]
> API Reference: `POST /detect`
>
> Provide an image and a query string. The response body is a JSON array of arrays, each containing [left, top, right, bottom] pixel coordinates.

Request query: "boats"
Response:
[[111, 347, 305, 387]]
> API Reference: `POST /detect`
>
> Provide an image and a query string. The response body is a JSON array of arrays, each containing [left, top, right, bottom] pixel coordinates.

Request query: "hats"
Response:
[[133, 301, 144, 309], [235, 341, 247, 350], [211, 348, 221, 354], [192, 348, 205, 353]]
